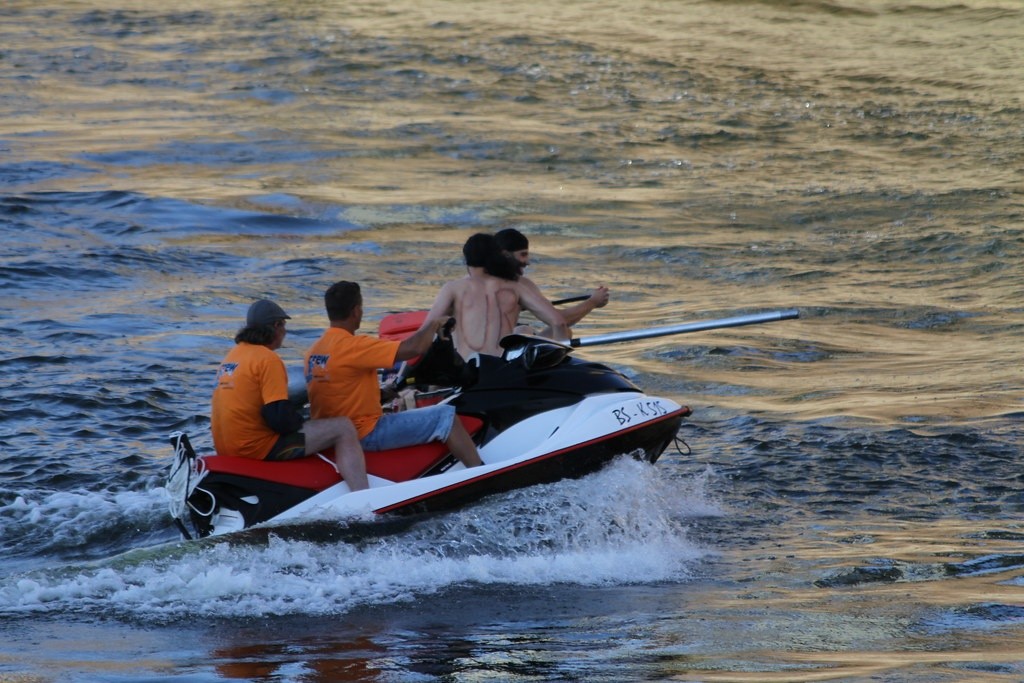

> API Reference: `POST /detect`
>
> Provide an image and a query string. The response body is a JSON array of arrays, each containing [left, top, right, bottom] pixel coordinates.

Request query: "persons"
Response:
[[211, 300, 369, 493], [418, 229, 610, 367], [303, 281, 486, 469]]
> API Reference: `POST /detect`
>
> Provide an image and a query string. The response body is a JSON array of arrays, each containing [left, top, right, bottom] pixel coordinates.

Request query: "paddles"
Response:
[[379, 295, 592, 343], [406, 307, 800, 366]]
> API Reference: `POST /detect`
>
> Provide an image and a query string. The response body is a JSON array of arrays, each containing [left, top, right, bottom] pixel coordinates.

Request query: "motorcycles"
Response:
[[164, 310, 694, 542]]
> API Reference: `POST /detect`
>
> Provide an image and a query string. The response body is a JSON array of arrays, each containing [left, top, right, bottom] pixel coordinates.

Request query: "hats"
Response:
[[247, 300, 291, 325], [495, 228, 528, 251]]
